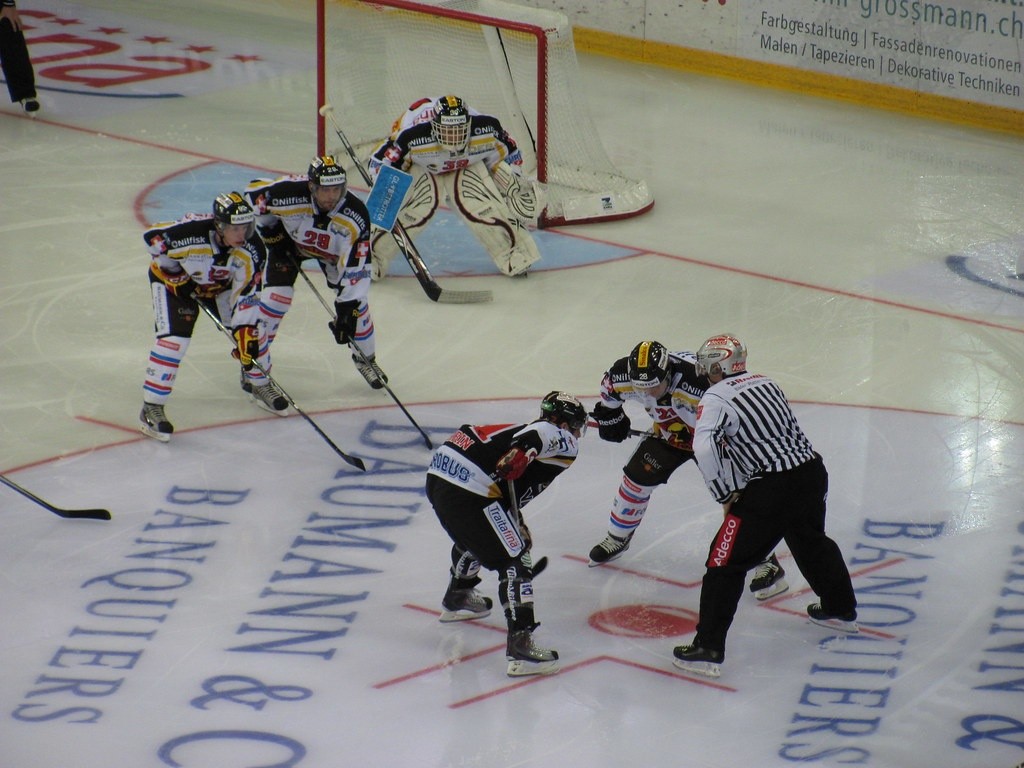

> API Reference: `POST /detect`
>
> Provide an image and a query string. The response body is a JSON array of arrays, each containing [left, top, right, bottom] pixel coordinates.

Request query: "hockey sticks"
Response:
[[189, 290, 367, 472], [0, 474, 111, 521], [505, 456, 548, 581], [319, 103, 494, 305], [587, 421, 666, 440], [294, 264, 433, 451]]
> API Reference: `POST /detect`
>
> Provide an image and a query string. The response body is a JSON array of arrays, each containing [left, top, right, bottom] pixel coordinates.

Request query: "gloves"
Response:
[[512, 510, 533, 551], [588, 401, 632, 443], [257, 220, 297, 264], [232, 319, 260, 372], [160, 266, 200, 302], [328, 299, 362, 344], [495, 447, 538, 481]]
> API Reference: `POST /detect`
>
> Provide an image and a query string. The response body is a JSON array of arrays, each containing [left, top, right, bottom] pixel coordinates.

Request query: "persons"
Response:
[[244, 154, 390, 396], [139, 191, 289, 444], [587, 341, 790, 600], [672, 334, 860, 677], [367, 95, 548, 281], [0, 0, 40, 118], [424, 391, 587, 676]]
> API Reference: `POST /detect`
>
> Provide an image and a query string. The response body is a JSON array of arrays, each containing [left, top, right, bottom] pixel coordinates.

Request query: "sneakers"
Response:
[[139, 402, 174, 443], [20, 97, 40, 119], [439, 567, 492, 622], [673, 643, 724, 677], [241, 366, 289, 417], [750, 552, 789, 600], [506, 622, 560, 677], [352, 354, 389, 397], [587, 529, 635, 568], [807, 603, 859, 633]]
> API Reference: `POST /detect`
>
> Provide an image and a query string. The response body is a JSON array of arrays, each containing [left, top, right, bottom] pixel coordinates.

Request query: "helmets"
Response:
[[696, 333, 748, 377], [540, 391, 587, 428], [430, 95, 471, 152], [308, 155, 346, 187], [628, 340, 670, 389], [213, 191, 255, 225]]
